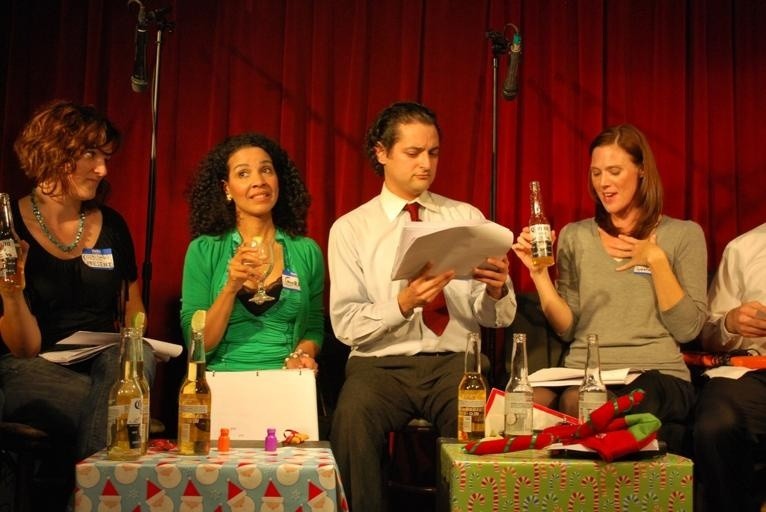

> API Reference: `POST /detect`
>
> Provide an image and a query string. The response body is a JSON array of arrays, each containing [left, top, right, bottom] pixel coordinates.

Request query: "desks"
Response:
[[73, 441, 350, 512], [437, 437, 696, 512]]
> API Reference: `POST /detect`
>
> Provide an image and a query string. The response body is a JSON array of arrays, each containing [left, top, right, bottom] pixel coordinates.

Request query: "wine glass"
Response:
[[242, 236, 278, 305]]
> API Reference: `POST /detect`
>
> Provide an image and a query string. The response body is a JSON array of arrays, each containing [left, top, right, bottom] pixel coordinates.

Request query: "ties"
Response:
[[401, 202, 451, 337]]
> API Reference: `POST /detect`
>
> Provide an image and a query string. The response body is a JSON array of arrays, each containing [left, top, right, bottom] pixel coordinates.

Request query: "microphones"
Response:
[[502, 33, 522, 100], [131, 7, 149, 92]]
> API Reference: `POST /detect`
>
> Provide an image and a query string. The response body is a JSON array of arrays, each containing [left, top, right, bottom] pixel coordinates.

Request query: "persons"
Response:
[[510, 122, 710, 423], [661, 221, 766, 512], [328, 83, 519, 511], [178, 130, 327, 376], [1, 98, 167, 511]]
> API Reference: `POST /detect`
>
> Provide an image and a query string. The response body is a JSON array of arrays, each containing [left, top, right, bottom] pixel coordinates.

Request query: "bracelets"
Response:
[[279, 347, 311, 370]]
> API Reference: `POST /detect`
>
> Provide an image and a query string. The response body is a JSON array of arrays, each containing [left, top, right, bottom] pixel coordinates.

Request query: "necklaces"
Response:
[[30, 184, 90, 252]]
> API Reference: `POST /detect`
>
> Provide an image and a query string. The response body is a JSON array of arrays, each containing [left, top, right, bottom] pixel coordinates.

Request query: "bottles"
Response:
[[1, 192, 22, 284], [457, 332, 487, 443], [528, 176, 556, 270], [265, 427, 277, 454], [217, 428, 232, 451], [107, 325, 151, 460], [579, 330, 609, 429], [173, 332, 213, 453], [503, 331, 535, 438]]
